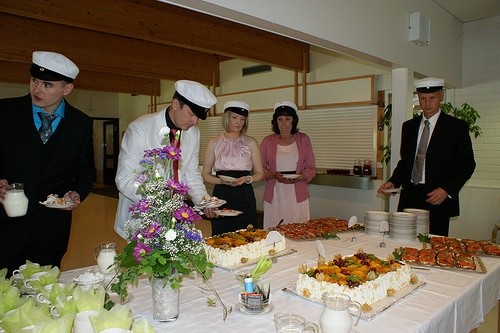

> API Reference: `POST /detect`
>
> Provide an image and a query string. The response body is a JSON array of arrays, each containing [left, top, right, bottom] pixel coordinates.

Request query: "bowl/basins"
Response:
[[235, 268, 265, 286], [237, 291, 271, 312]]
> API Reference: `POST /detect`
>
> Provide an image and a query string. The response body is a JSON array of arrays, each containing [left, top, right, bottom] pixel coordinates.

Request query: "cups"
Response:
[[13, 263, 128, 333]]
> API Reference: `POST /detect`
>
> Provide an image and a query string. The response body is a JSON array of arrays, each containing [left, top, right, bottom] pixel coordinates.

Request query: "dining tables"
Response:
[[0, 219, 500, 333]]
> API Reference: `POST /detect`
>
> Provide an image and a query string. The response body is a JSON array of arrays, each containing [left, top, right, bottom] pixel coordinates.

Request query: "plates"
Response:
[[213, 210, 244, 216], [39, 198, 76, 208], [364, 207, 431, 244], [217, 175, 238, 181], [194, 199, 226, 209], [282, 174, 303, 179], [382, 188, 402, 193]]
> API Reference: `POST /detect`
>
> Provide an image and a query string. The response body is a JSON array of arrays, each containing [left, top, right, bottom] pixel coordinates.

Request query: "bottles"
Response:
[[352, 159, 372, 176]]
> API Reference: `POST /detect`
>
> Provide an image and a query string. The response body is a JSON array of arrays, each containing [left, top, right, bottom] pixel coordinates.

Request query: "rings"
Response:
[[73, 196, 79, 199]]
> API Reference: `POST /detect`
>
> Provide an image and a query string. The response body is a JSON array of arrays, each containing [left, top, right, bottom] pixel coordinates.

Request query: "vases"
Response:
[[150, 266, 181, 322]]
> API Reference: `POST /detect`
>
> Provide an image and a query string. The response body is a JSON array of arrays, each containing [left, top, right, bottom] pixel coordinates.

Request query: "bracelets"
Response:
[[245, 176, 254, 185]]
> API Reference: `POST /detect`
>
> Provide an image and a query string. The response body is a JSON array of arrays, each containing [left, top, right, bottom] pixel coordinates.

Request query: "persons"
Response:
[[202, 101, 264, 239], [0, 51, 95, 281], [377, 76, 477, 240], [111, 80, 224, 257], [256, 100, 319, 230]]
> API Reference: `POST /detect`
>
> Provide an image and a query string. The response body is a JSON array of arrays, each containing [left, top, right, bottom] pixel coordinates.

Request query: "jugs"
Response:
[[1, 183, 29, 217], [94, 241, 117, 274], [274, 313, 318, 333], [319, 291, 362, 333]]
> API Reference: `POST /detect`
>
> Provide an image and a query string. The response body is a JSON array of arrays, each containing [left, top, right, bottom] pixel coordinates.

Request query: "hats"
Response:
[[416, 77, 444, 92], [274, 101, 298, 115], [30, 51, 79, 83], [174, 80, 217, 120], [224, 101, 249, 117]]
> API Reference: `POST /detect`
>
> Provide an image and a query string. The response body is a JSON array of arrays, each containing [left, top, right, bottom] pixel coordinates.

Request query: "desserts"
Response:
[[195, 194, 219, 206], [44, 194, 66, 205]]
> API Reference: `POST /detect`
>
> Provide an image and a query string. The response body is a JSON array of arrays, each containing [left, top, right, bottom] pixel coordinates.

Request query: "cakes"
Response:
[[296, 247, 418, 312], [201, 224, 286, 266]]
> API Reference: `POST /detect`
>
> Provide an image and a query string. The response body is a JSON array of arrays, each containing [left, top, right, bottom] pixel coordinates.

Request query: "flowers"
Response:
[[104, 130, 221, 306]]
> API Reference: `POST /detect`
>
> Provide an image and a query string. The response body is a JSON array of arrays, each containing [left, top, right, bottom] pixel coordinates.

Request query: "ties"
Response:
[[171, 128, 178, 184], [412, 120, 431, 185], [37, 112, 59, 145]]
